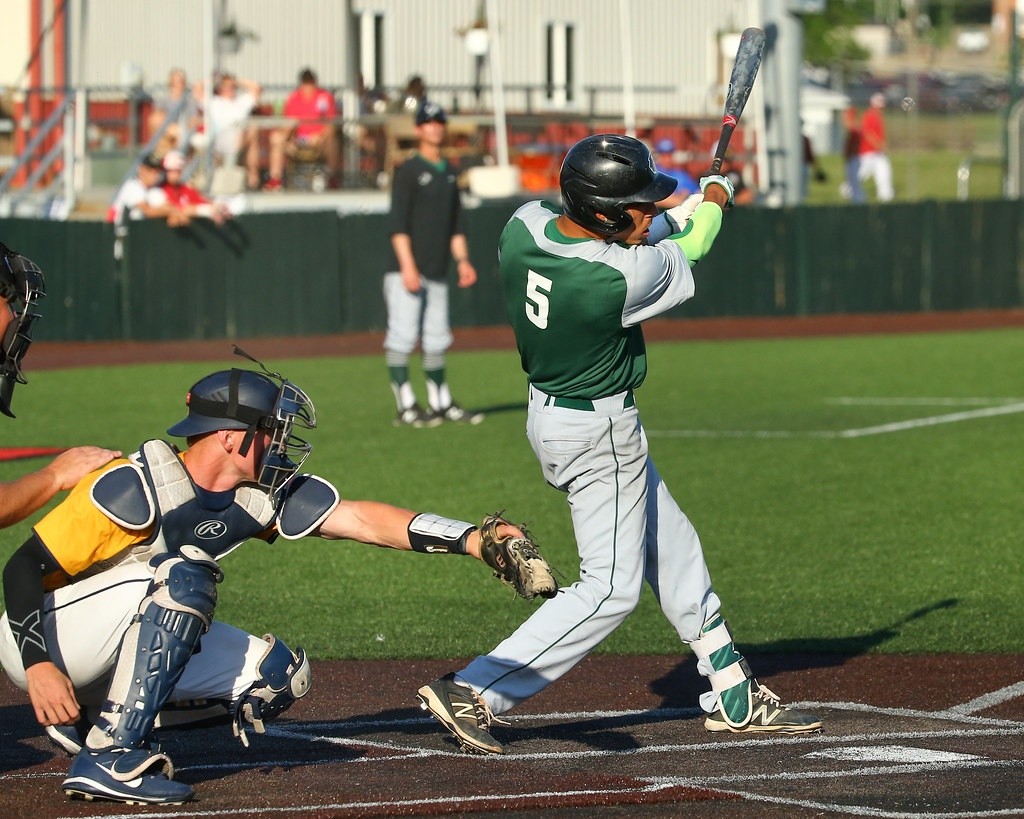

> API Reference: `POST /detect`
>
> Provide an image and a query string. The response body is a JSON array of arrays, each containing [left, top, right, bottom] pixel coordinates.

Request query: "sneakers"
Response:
[[393, 403, 442, 427], [426, 401, 485, 425], [416, 672, 512, 755], [704, 678, 825, 734], [62, 744, 193, 805], [46, 725, 83, 754]]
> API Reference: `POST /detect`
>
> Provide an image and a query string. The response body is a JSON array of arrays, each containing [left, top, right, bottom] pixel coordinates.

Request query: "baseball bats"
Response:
[[705, 26, 768, 176]]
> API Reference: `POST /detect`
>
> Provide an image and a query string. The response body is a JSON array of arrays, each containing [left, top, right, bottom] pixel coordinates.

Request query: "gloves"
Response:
[[699, 174, 735, 208], [665, 193, 705, 234]]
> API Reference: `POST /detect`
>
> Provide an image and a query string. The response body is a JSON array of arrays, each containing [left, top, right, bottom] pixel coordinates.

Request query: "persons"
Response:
[[755, 106, 827, 191], [190, 72, 265, 193], [149, 68, 205, 156], [840, 93, 895, 200], [416, 132, 824, 755], [380, 105, 485, 428], [0, 367, 560, 807], [264, 66, 340, 191], [652, 136, 701, 206], [0, 244, 122, 528], [695, 140, 755, 205], [108, 152, 230, 229]]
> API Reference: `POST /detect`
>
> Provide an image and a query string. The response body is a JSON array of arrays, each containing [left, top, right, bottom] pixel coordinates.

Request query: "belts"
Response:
[[545, 389, 634, 412]]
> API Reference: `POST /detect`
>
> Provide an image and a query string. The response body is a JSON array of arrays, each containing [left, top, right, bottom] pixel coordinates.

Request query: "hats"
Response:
[[142, 151, 164, 170], [163, 152, 188, 172], [413, 102, 448, 127]]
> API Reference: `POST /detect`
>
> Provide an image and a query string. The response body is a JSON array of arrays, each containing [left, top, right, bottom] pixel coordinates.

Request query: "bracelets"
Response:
[[453, 256, 468, 267], [196, 203, 214, 217]]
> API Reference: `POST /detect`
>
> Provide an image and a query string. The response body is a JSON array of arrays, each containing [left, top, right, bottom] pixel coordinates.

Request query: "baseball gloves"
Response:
[[478, 509, 567, 601]]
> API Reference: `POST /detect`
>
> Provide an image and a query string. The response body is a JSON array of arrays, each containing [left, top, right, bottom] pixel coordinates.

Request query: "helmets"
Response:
[[167, 369, 292, 437], [559, 134, 679, 235]]
